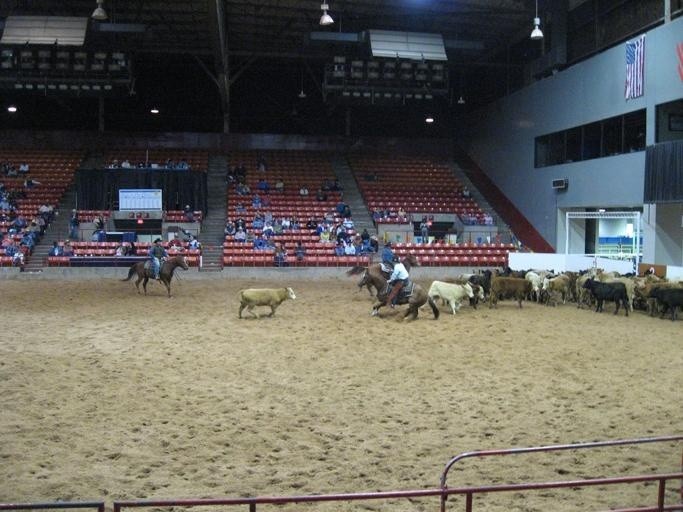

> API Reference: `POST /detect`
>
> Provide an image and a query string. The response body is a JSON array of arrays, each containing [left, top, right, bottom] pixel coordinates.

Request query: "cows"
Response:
[[238, 286, 296, 319], [421, 266, 683, 316]]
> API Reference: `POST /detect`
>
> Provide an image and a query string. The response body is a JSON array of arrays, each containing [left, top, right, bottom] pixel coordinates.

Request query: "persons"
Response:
[[0, 160, 202, 280], [387, 256, 410, 308], [223, 157, 494, 267]]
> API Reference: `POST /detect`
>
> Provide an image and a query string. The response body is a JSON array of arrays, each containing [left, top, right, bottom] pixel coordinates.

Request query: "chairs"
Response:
[[0, 149, 208, 266], [223, 151, 519, 266]]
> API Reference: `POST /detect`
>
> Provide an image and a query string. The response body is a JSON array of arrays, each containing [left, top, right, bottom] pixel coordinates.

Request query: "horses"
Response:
[[346, 253, 422, 297], [119, 255, 190, 300], [356, 267, 440, 321]]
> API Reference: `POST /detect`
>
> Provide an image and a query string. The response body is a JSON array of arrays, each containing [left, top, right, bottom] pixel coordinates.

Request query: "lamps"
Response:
[[298, 69, 306, 98], [457, 97, 465, 104], [530, 0, 543, 40], [319, 0, 335, 26], [91, 0, 109, 20]]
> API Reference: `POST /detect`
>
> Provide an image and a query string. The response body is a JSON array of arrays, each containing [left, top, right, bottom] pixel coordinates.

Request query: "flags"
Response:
[[624, 34, 645, 101]]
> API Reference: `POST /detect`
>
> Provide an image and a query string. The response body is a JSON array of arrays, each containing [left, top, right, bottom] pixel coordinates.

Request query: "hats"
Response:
[[391, 257, 399, 263], [20, 242, 26, 245], [154, 238, 161, 243], [64, 240, 69, 245]]
